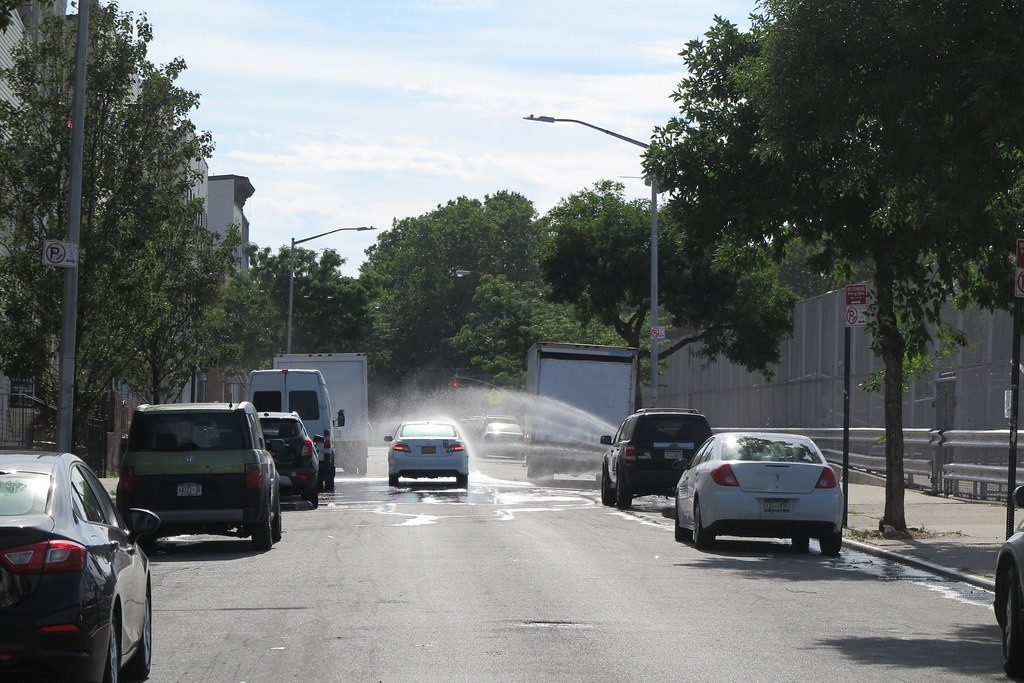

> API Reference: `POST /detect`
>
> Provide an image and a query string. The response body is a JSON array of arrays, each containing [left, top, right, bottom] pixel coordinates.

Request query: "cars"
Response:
[[671, 431, 845, 555], [253, 410, 320, 509], [383, 420, 469, 488], [0, 450, 161, 683], [460, 414, 530, 460], [992, 484, 1024, 679]]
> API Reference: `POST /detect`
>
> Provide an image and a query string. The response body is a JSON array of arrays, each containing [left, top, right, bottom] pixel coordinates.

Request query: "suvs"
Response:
[[116, 400, 283, 550], [601, 407, 714, 509]]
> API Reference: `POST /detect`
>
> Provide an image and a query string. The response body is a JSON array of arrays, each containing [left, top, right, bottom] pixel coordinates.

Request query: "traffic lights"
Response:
[[451, 381, 463, 393], [447, 383, 455, 402]]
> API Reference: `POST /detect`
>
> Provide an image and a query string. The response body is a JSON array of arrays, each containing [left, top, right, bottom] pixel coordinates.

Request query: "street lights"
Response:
[[521, 113, 665, 407], [287, 225, 378, 355]]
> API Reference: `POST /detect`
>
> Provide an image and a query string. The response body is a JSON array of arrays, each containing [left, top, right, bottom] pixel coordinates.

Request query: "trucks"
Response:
[[272, 352, 368, 476], [525, 342, 641, 486]]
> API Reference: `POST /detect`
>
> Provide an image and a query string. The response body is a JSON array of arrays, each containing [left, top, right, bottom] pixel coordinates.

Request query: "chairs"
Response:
[[675, 423, 697, 438], [278, 424, 293, 437], [725, 447, 752, 460], [155, 433, 178, 449], [220, 432, 239, 448], [784, 447, 811, 463]]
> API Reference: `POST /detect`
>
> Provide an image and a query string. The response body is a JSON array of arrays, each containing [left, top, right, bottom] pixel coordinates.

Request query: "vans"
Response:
[[237, 368, 346, 494]]
